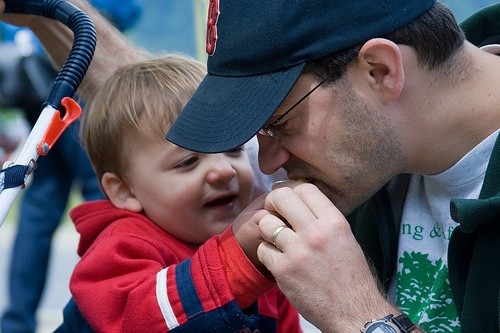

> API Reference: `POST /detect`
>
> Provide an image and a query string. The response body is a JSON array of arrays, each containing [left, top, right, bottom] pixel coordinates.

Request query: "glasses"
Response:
[[256, 55, 358, 145]]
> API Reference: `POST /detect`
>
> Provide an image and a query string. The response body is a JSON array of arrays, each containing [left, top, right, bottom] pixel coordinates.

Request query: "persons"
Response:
[[0, 0, 500, 333], [55, 54, 303, 333], [0, 1, 141, 333]]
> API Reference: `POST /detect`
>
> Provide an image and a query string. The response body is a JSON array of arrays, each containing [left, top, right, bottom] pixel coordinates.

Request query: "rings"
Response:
[[272, 224, 289, 246]]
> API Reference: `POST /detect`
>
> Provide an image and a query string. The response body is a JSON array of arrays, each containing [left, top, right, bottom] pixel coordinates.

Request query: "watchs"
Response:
[[359, 314, 416, 333]]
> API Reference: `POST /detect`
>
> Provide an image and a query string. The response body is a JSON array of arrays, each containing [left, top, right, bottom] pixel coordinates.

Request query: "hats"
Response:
[[163, 1, 437, 155]]
